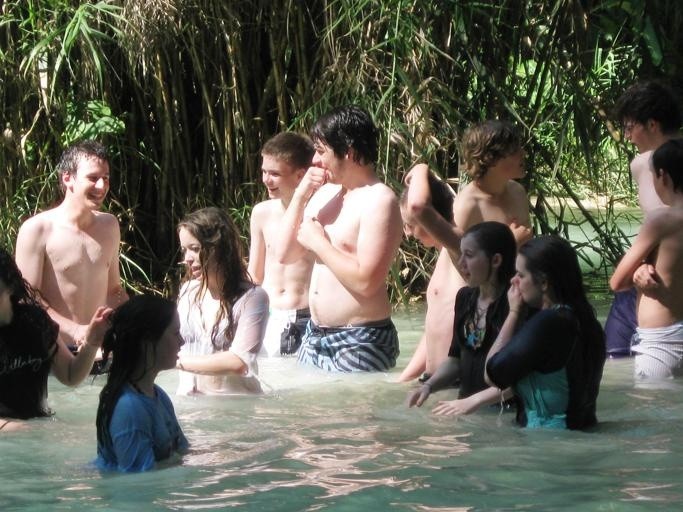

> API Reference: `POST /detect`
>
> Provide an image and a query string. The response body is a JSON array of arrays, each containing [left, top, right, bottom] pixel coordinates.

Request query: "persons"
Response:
[[604, 84, 683, 358], [0, 246, 112, 430], [483, 235, 606, 432], [407, 222, 516, 416], [246, 132, 315, 357], [453, 120, 532, 245], [18, 145, 130, 375], [277, 105, 401, 374], [175, 206, 269, 396], [94, 294, 189, 474], [609, 139, 683, 385], [399, 164, 466, 379]]
